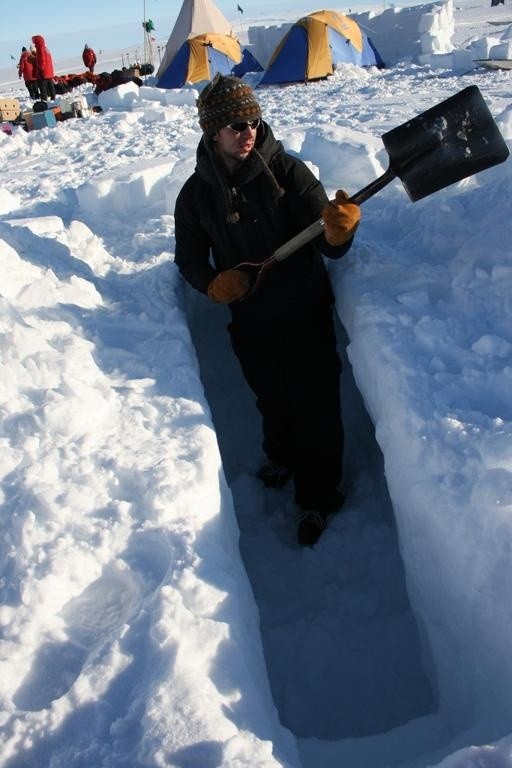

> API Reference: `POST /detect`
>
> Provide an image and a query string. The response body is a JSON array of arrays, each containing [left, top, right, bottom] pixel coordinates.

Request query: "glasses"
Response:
[[227, 118, 261, 133]]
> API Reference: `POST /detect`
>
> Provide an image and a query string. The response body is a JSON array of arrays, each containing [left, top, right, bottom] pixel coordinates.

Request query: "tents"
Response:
[[255, 8, 387, 86], [155, 0, 264, 90]]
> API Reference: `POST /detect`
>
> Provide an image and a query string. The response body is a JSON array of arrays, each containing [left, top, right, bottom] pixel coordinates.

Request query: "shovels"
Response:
[[232, 85, 510, 298]]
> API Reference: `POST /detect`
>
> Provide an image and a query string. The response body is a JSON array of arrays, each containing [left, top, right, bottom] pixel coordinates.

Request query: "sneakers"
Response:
[[296, 510, 325, 546], [256, 464, 293, 490]]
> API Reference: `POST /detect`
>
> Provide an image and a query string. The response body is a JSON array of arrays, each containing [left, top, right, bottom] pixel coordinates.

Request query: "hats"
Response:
[[21, 46, 28, 52], [195, 72, 262, 140], [85, 43, 89, 50]]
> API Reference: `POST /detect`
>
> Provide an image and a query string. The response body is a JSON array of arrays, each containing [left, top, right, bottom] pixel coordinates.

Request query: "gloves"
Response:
[[322, 190, 361, 246], [207, 270, 252, 305]]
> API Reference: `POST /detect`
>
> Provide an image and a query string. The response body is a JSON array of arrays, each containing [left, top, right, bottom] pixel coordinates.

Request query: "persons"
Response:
[[27, 35, 56, 104], [16, 46, 40, 101], [82, 43, 97, 74], [174, 69, 369, 549]]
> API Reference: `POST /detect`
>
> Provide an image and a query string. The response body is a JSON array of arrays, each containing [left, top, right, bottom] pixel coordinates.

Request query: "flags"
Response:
[[237, 5, 244, 14]]
